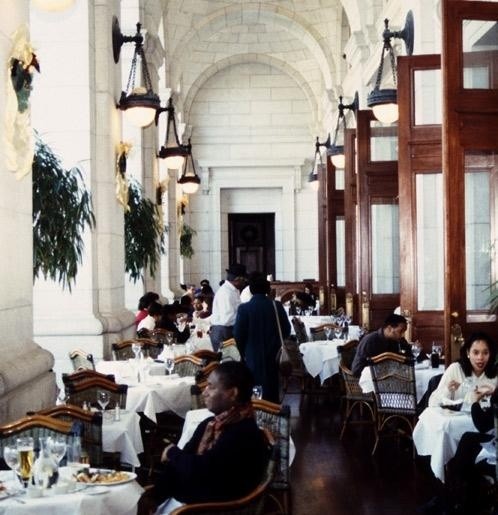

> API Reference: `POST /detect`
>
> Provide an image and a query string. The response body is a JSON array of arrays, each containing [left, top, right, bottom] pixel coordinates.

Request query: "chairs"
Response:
[[164, 426, 282, 515], [67, 348, 95, 374], [250, 396, 293, 513], [65, 379, 127, 419], [334, 339, 360, 364], [113, 339, 142, 362], [290, 315, 311, 346], [138, 336, 165, 360], [135, 327, 177, 346], [308, 324, 347, 343], [0, 414, 93, 471], [61, 369, 115, 389], [189, 350, 222, 370], [194, 361, 223, 381], [189, 375, 208, 410], [218, 337, 246, 362], [363, 351, 421, 460], [280, 335, 309, 411], [26, 404, 104, 463], [338, 362, 384, 442], [166, 355, 206, 381]]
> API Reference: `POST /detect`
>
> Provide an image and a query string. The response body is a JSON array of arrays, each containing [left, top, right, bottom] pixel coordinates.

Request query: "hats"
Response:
[[225, 265, 249, 276]]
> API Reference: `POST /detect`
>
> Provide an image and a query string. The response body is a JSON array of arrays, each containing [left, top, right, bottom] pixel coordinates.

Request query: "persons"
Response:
[[135, 358, 271, 515], [134, 302, 164, 332], [178, 279, 214, 317], [209, 262, 250, 354], [351, 314, 415, 378], [290, 283, 320, 316], [427, 331, 498, 412], [423, 382, 498, 515], [132, 292, 160, 325], [174, 312, 190, 345], [234, 272, 292, 405]]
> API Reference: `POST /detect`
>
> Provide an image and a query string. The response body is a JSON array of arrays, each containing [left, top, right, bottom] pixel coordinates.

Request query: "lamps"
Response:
[[176, 137, 200, 197], [157, 95, 186, 172], [306, 132, 331, 191], [366, 9, 415, 125], [330, 90, 360, 171], [111, 16, 160, 130]]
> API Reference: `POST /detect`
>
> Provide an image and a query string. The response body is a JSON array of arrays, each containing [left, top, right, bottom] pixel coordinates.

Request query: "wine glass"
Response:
[[305, 306, 312, 321], [165, 358, 173, 377], [252, 385, 262, 399], [334, 328, 342, 343], [324, 326, 331, 345], [165, 332, 173, 345], [297, 306, 301, 318], [47, 439, 66, 471], [97, 391, 109, 415], [132, 343, 141, 360], [433, 346, 443, 359], [412, 344, 421, 362], [4, 446, 22, 485]]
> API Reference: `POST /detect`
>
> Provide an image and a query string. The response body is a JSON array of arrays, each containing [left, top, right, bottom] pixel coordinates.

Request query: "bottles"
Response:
[[431, 349, 440, 369], [33, 435, 59, 492]]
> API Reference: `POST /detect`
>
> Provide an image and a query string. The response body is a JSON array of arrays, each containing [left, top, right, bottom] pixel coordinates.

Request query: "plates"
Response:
[[67, 468, 137, 484], [82, 486, 110, 495]]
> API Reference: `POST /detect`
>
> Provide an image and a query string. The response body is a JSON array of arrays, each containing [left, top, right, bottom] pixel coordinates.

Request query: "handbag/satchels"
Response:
[[273, 300, 296, 376]]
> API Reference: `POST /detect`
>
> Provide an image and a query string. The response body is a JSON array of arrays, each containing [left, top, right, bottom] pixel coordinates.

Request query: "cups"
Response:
[[17, 438, 35, 478], [52, 484, 67, 494], [27, 487, 41, 497]]
